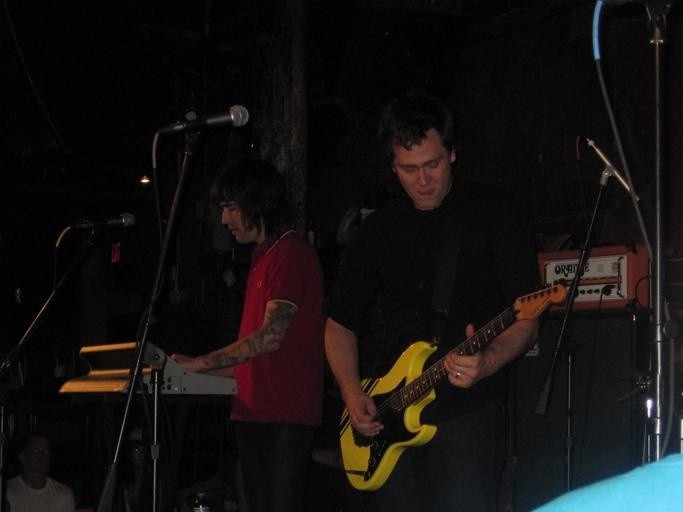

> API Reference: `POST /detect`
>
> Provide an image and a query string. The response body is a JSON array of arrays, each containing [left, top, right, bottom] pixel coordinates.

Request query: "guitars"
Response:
[[340, 284, 568, 491]]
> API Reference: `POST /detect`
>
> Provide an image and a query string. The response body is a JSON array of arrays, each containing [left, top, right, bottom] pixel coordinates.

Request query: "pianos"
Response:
[[59, 343, 238, 395]]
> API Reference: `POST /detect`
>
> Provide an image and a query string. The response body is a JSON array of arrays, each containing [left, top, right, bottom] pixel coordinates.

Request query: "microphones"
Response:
[[68, 212, 135, 231], [166, 104, 251, 135], [583, 137, 641, 203]]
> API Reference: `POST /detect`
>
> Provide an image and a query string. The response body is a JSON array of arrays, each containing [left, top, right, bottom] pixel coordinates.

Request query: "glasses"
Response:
[[23, 447, 50, 456]]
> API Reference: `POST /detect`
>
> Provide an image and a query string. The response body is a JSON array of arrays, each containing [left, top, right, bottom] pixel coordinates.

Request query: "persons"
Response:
[[171, 153, 324, 512], [5, 432, 76, 512], [323, 97, 539, 511], [115, 428, 176, 512]]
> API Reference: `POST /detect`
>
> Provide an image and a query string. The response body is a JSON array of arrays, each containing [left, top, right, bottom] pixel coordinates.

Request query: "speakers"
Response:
[[505, 306, 649, 511]]
